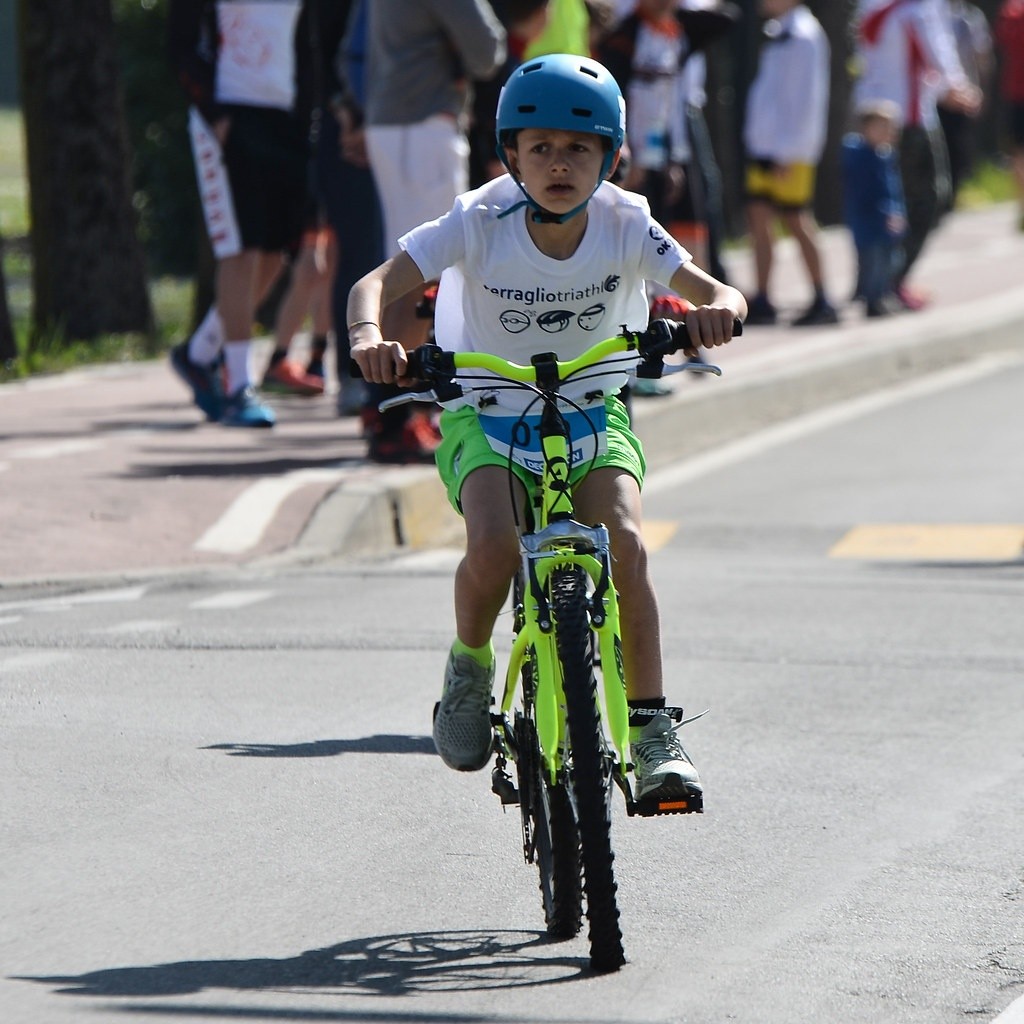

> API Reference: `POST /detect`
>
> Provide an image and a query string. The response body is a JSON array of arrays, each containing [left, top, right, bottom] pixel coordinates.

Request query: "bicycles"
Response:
[[346, 300, 741, 972]]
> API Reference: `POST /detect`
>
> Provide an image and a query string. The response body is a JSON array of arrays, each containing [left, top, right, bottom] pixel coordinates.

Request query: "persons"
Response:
[[159, 1, 1019, 464], [346, 52, 749, 813]]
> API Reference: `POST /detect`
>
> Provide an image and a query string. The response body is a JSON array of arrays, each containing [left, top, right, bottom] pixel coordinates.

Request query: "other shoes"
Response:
[[634, 379, 670, 397], [790, 303, 838, 324], [338, 381, 369, 416], [865, 299, 888, 315], [264, 361, 325, 394], [893, 284, 924, 309], [745, 298, 776, 323]]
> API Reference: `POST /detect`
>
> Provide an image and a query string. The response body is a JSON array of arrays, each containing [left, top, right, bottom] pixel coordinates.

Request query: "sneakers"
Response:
[[363, 405, 442, 464], [631, 714, 704, 813], [433, 650, 497, 771], [173, 341, 276, 426]]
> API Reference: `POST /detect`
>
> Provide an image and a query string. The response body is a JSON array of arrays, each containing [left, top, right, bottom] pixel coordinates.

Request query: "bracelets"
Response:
[[348, 321, 379, 338]]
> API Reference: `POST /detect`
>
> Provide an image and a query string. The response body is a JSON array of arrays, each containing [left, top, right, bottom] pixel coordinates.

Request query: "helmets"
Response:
[[496, 55, 627, 225]]
